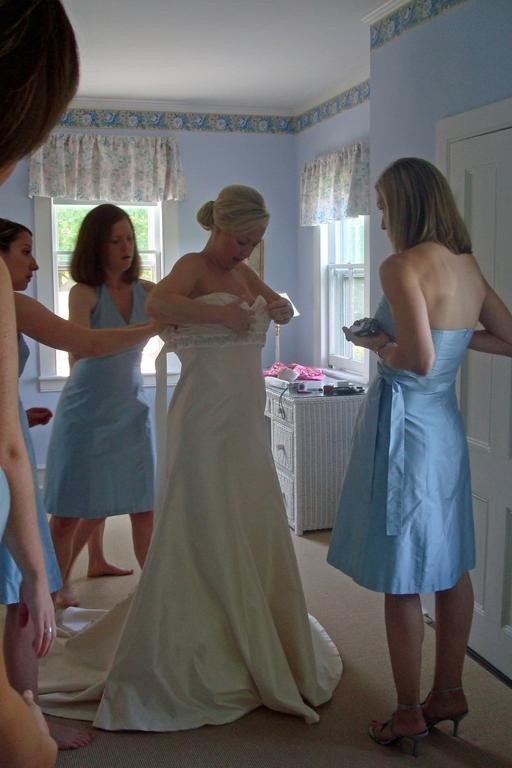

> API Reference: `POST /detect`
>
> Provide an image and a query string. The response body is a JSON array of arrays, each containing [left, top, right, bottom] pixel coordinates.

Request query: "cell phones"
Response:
[[349, 317, 378, 337]]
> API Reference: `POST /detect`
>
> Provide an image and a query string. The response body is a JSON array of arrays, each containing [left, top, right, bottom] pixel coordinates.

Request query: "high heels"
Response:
[[367, 703, 430, 758], [421, 686, 469, 738]]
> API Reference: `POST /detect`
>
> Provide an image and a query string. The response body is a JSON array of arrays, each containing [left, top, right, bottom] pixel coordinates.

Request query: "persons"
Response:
[[143, 186, 342, 733], [341, 157, 512, 756]]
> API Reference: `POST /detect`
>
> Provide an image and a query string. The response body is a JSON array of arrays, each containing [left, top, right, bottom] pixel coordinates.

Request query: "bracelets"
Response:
[[376, 341, 396, 359]]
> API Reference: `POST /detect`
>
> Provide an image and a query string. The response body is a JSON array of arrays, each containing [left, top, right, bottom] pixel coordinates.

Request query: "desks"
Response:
[[263, 367, 368, 537]]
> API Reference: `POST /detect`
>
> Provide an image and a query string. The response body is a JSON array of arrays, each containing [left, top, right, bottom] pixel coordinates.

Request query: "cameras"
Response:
[[288, 382, 307, 393]]
